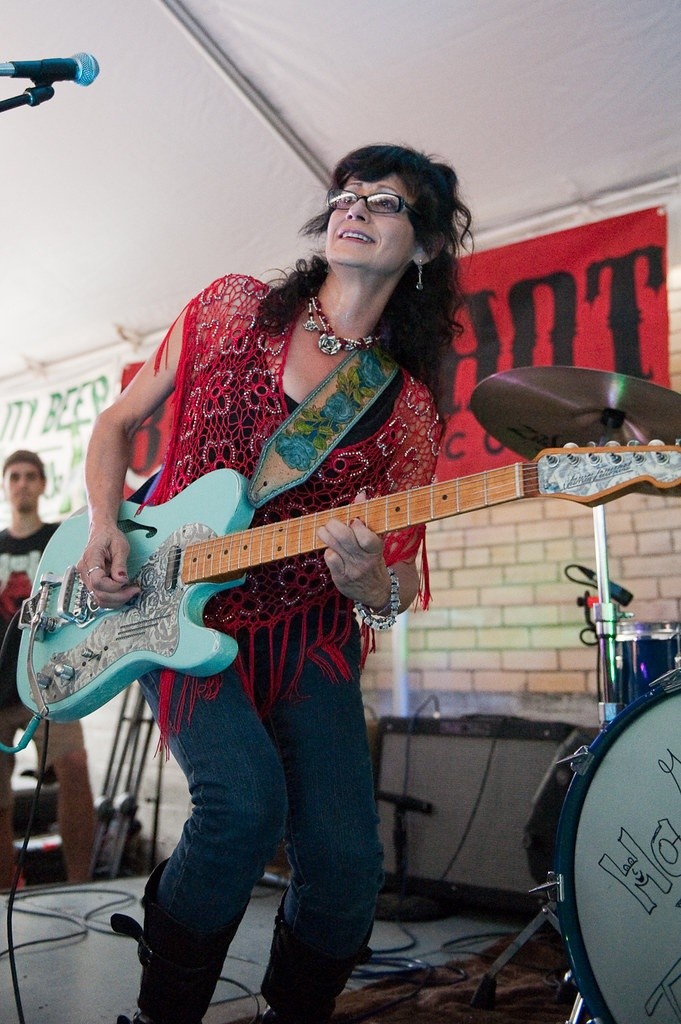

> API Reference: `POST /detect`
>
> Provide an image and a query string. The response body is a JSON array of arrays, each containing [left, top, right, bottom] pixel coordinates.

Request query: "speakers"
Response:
[[372, 714, 594, 918]]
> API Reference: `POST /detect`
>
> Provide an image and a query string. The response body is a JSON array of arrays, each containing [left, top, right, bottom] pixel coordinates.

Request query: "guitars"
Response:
[[13, 435, 681, 728]]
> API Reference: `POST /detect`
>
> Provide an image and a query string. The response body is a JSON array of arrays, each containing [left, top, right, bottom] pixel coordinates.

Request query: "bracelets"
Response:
[[354, 565, 401, 631]]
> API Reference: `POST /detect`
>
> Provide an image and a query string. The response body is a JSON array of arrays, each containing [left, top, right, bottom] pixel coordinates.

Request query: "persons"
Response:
[[74, 145, 472, 1024], [0, 450, 97, 890]]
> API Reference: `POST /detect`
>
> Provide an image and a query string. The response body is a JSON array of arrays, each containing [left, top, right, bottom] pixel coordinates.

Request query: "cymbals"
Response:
[[469, 362, 681, 462]]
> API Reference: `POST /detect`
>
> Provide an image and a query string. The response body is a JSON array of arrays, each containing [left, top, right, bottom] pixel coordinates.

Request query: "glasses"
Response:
[[326, 188, 421, 214]]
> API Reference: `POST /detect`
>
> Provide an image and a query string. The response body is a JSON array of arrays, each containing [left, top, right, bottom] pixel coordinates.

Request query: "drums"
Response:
[[616, 620, 681, 709], [554, 669, 681, 1024]]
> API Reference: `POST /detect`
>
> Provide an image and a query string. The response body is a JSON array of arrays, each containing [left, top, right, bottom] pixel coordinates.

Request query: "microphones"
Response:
[[0, 52, 100, 87], [577, 566, 633, 606], [375, 790, 433, 815]]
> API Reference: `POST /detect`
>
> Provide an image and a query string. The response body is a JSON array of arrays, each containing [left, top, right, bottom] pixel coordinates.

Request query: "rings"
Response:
[[86, 566, 100, 576], [87, 591, 95, 599]]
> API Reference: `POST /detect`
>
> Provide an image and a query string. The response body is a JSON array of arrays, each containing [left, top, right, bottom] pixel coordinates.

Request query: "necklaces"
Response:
[[302, 295, 382, 355]]
